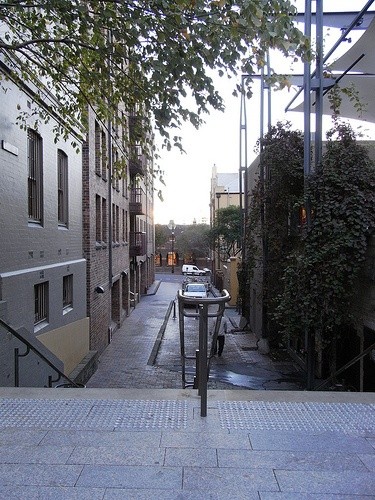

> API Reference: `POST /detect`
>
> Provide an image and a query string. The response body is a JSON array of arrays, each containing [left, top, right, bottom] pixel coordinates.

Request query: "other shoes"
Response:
[[212, 353, 221, 357]]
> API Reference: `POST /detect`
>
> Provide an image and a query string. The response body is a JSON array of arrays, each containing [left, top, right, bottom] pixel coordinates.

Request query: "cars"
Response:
[[182, 283, 208, 306]]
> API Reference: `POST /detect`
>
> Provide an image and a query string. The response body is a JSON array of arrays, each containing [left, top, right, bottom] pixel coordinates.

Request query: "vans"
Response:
[[182, 264, 205, 275]]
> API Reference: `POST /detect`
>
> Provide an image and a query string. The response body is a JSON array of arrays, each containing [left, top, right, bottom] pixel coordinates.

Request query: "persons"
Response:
[[208, 316, 228, 358]]
